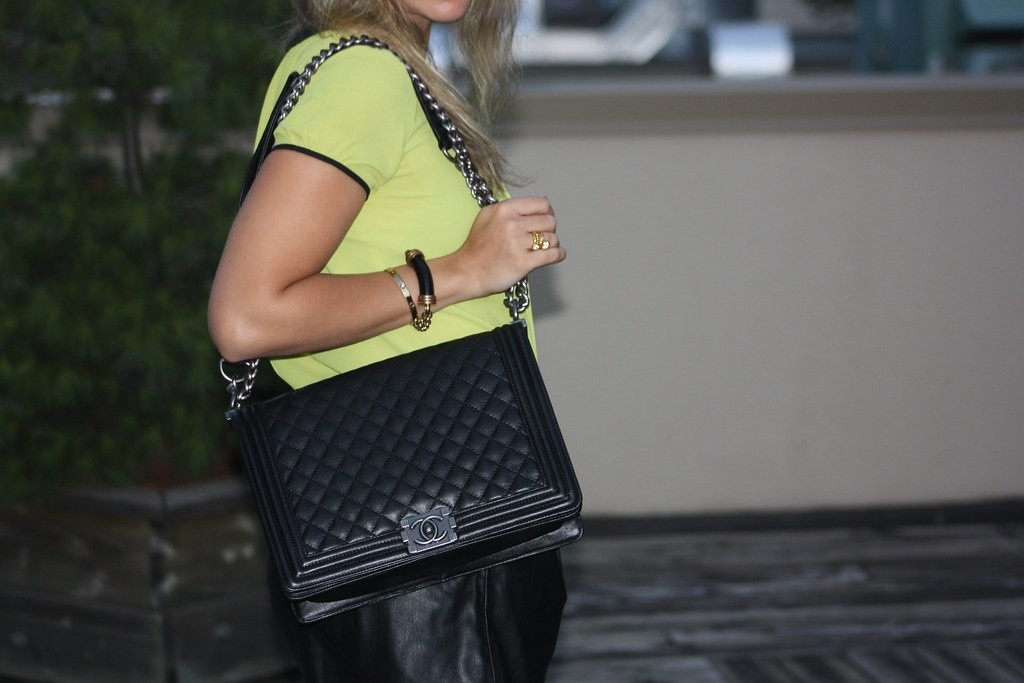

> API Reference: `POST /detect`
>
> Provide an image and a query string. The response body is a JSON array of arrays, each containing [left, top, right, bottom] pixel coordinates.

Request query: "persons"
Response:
[[206, 0, 567, 683]]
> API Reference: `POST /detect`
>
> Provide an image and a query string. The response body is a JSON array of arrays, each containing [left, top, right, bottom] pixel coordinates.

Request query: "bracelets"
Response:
[[405, 249, 436, 331], [385, 268, 417, 321]]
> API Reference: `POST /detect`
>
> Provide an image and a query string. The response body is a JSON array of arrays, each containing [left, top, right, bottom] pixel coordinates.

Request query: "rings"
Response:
[[530, 231, 549, 251]]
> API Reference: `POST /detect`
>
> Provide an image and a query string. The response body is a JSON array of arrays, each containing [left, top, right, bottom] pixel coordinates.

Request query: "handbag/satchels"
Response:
[[230, 323, 585, 627]]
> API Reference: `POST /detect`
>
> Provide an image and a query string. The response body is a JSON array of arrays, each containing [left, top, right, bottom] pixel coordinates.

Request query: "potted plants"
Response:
[[0, 1, 312, 683]]
